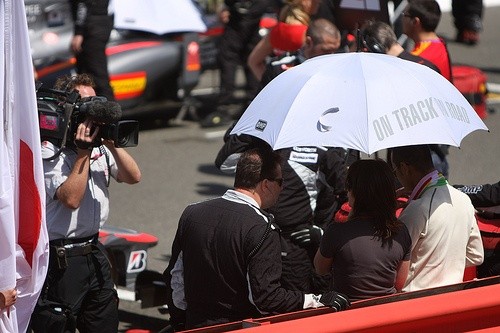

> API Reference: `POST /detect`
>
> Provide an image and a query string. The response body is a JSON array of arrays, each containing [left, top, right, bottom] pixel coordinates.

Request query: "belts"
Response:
[[50, 236, 114, 268]]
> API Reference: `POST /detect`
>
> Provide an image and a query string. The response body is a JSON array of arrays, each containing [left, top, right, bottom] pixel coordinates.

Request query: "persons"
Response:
[[452, 180, 500, 279], [313, 160, 412, 300], [66, 0, 115, 101], [163, 149, 352, 330], [0, 288, 17, 311], [451, 0, 483, 45], [200, 0, 454, 177], [390, 145, 484, 293], [31, 73, 142, 333], [271, 146, 348, 295]]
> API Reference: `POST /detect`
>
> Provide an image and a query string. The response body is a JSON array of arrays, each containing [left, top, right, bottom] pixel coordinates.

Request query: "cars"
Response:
[[28, 12, 224, 127]]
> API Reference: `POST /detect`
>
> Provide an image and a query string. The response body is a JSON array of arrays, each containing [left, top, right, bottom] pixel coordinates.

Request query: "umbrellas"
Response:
[[108, 0, 207, 36], [229, 52, 491, 158]]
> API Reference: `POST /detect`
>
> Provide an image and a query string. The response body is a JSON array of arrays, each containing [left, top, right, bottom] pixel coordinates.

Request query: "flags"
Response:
[[0, 1, 50, 333]]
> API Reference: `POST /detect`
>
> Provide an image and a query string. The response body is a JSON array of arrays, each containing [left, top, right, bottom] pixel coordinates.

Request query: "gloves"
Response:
[[319, 291, 352, 312]]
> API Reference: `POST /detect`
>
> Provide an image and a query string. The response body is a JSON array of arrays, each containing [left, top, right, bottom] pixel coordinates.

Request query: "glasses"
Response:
[[392, 166, 399, 176], [402, 11, 414, 18], [271, 178, 284, 186]]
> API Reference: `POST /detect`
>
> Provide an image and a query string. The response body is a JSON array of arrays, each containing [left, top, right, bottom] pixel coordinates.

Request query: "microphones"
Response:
[[80, 101, 122, 123]]
